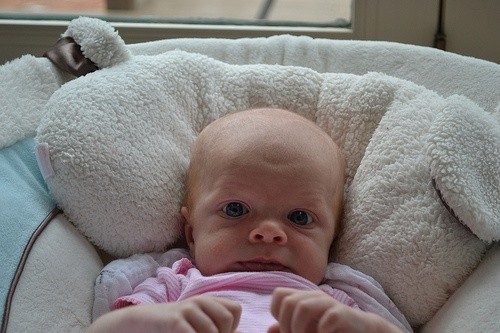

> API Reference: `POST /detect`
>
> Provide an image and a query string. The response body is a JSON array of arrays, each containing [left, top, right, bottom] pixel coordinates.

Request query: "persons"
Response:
[[84, 107, 402, 333]]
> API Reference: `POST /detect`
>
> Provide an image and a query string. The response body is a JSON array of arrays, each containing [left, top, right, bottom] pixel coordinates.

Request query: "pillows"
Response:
[[35, 17, 500, 327]]
